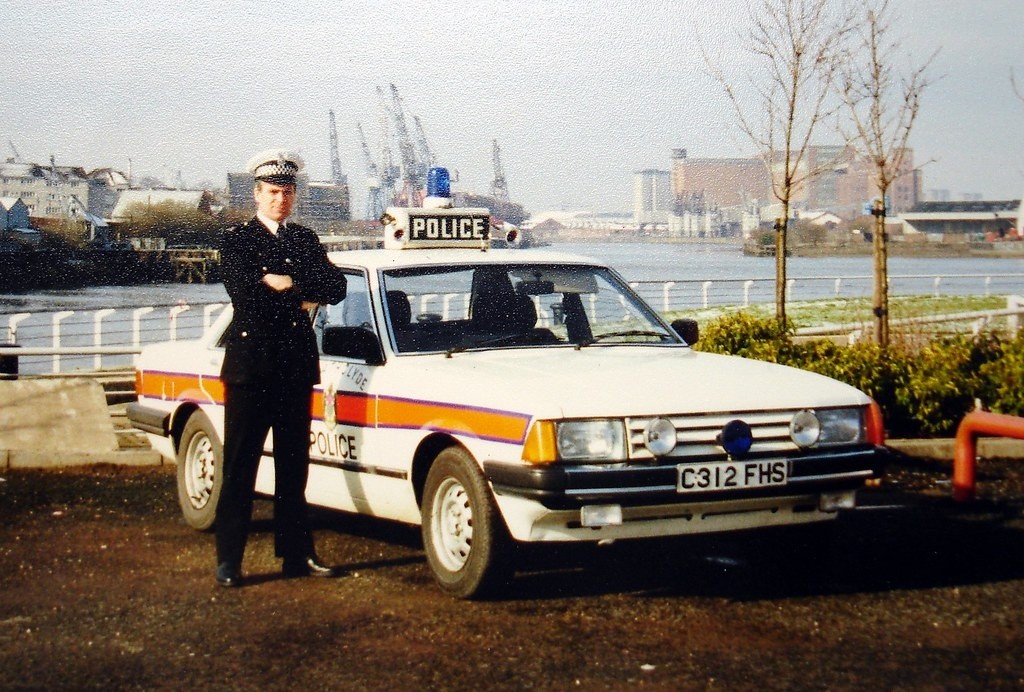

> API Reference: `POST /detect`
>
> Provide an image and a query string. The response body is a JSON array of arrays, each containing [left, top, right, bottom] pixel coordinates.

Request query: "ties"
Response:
[[277, 224, 286, 243]]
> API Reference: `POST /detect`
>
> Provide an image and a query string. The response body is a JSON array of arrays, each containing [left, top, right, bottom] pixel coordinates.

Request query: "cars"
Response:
[[124, 166, 889, 598]]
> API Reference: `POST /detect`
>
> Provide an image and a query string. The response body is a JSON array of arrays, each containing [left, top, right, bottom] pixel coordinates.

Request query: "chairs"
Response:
[[387, 290, 425, 352], [481, 294, 560, 345]]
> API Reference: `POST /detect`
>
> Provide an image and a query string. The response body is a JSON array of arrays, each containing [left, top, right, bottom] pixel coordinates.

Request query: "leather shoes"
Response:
[[215, 560, 244, 587], [283, 551, 340, 578]]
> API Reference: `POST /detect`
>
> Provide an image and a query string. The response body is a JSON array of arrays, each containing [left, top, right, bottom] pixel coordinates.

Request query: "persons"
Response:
[[211, 146, 350, 586]]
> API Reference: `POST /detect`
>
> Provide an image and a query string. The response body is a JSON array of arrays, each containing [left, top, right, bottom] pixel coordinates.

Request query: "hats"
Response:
[[247, 149, 305, 185]]
